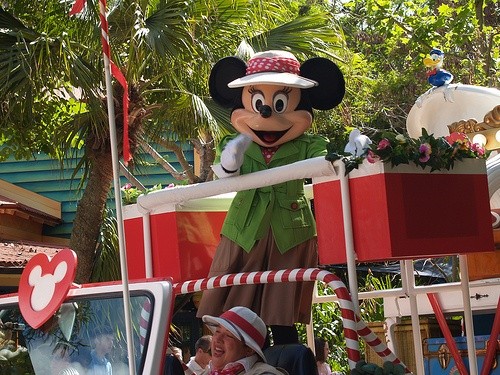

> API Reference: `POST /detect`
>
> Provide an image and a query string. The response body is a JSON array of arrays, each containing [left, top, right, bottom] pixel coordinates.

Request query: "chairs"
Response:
[[263, 345, 319, 375]]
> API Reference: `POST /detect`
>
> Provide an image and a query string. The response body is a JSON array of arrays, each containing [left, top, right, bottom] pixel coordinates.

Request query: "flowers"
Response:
[[344, 128, 490, 173], [120, 183, 174, 205]]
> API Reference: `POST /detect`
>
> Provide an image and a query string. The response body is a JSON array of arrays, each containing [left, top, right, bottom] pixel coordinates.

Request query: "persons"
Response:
[[47, 306, 333, 375], [195, 49, 372, 347]]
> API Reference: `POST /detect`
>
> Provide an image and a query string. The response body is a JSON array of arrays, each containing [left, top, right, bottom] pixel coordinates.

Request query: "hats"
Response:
[[202, 305, 270, 363]]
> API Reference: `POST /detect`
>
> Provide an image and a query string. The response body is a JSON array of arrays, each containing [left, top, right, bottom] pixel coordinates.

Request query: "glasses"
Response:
[[203, 348, 213, 357]]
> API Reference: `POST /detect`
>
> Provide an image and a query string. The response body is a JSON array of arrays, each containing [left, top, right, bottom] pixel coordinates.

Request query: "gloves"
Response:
[[208, 130, 254, 181]]
